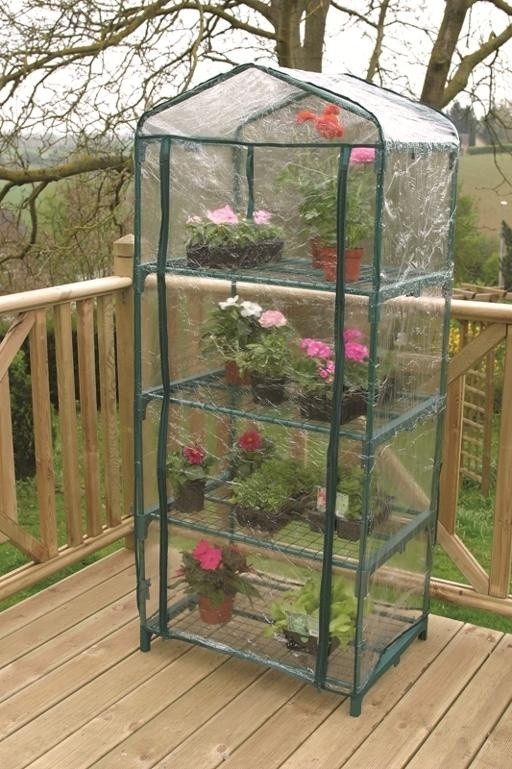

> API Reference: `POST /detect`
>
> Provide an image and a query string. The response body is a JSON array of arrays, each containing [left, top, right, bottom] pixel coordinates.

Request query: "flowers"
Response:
[[195, 295, 272, 360], [322, 146, 379, 252], [166, 443, 220, 491], [166, 538, 265, 608], [221, 428, 274, 473], [272, 97, 348, 240], [292, 329, 381, 400], [181, 207, 281, 249], [240, 310, 302, 376]]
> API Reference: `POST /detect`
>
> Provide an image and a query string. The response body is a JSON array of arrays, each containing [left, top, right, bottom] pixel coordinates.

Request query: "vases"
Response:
[[309, 236, 332, 268], [297, 376, 394, 424], [186, 235, 283, 272], [223, 358, 253, 385], [173, 479, 206, 511], [320, 245, 366, 284], [249, 372, 286, 404], [198, 594, 234, 624]]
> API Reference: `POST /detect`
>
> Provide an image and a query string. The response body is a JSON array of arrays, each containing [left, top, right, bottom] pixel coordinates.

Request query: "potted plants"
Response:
[[228, 460, 313, 532], [305, 462, 348, 531], [264, 569, 371, 656], [336, 465, 394, 536]]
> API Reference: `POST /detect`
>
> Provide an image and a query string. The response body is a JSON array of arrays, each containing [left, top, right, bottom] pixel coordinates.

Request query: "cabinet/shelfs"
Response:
[[125, 62, 460, 715]]
